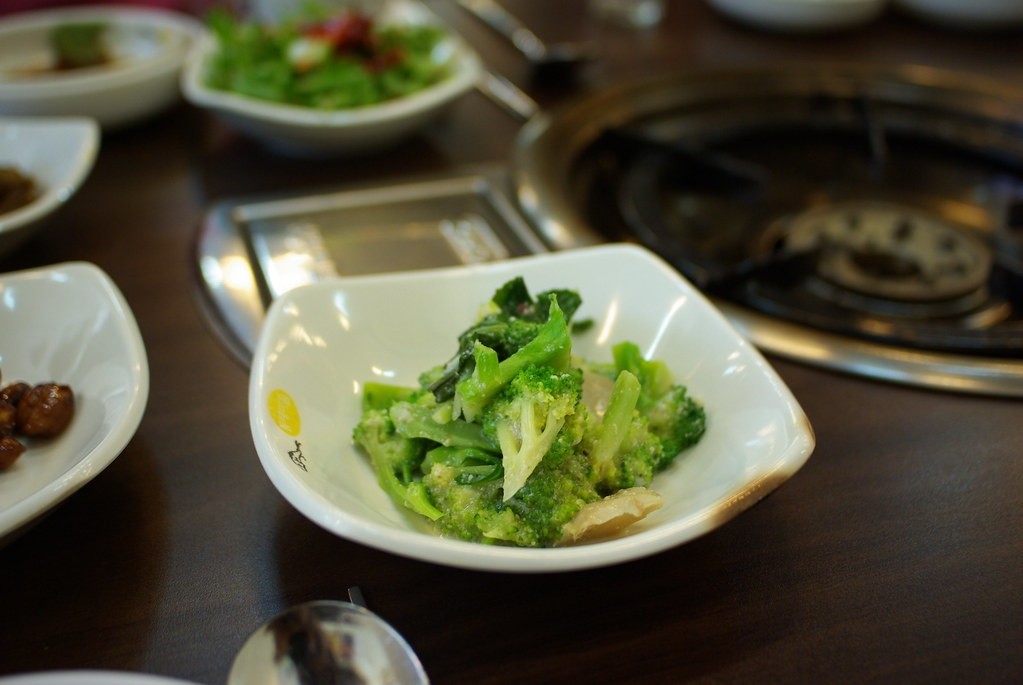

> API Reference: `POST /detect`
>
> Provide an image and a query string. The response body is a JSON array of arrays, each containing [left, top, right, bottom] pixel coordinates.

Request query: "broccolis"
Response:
[[349, 277, 708, 546]]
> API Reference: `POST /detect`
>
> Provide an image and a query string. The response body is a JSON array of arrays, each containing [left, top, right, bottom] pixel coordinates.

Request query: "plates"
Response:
[[0, 261, 150, 535], [181, 1, 482, 157], [247, 244, 816, 572], [0, 10, 204, 123], [0, 117, 100, 235]]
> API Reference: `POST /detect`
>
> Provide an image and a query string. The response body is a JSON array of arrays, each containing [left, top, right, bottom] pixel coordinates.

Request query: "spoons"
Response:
[[222, 598, 426, 685]]
